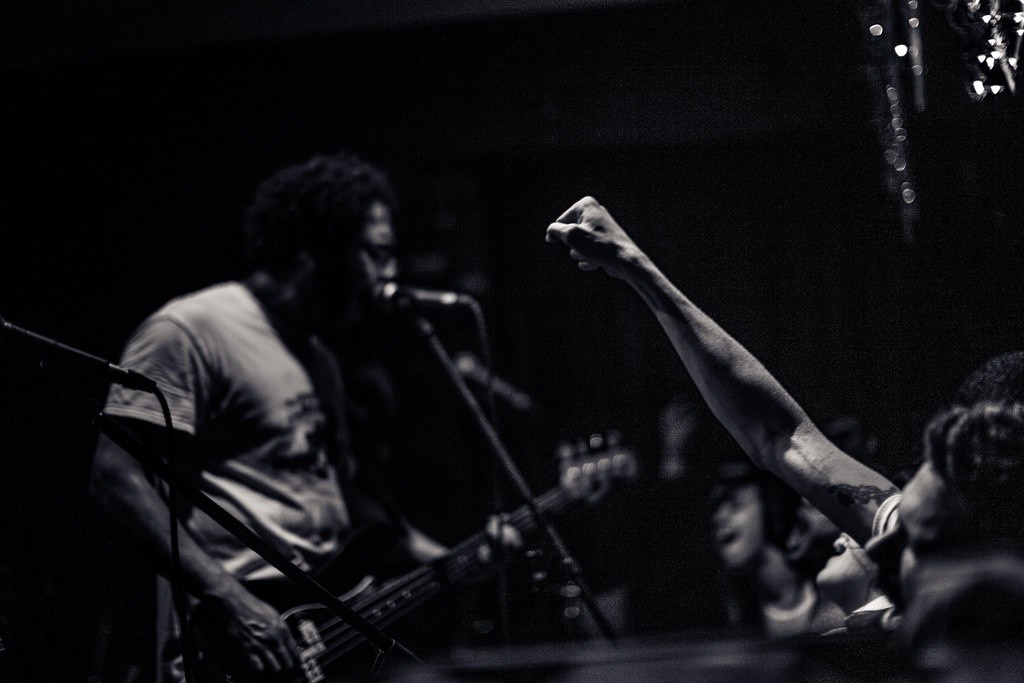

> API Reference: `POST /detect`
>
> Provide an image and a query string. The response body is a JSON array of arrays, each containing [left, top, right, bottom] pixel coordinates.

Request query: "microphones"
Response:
[[0, 321, 157, 392], [384, 282, 468, 307], [458, 356, 539, 414]]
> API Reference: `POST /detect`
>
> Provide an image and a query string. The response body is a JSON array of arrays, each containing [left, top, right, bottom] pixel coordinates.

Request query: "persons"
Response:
[[546, 197, 1024, 683], [618, 350, 1024, 638], [94, 157, 522, 683]]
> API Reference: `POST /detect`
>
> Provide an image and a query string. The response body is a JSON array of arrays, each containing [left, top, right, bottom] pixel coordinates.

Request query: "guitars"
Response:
[[182, 431, 639, 683]]
